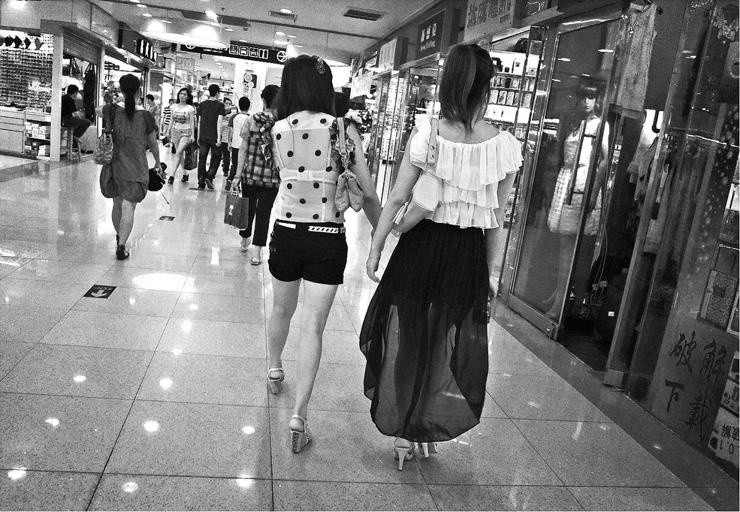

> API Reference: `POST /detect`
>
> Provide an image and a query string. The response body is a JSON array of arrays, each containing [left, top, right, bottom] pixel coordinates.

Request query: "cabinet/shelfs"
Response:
[[486, 70, 537, 229], [0, 105, 51, 161]]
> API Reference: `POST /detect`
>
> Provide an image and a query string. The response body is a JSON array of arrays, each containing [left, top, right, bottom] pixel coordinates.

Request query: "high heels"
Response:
[[418, 443, 437, 457], [393, 437, 414, 470], [290, 415, 312, 453], [268, 368, 284, 394]]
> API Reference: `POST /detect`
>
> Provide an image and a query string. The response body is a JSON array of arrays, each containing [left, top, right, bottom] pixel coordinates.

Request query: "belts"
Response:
[[279, 221, 346, 234]]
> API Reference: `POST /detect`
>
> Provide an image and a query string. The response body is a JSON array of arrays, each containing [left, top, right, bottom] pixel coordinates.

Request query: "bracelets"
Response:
[[234, 176, 241, 178]]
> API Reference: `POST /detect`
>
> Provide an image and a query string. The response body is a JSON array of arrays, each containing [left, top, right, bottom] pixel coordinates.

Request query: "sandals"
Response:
[[116, 245, 129, 260]]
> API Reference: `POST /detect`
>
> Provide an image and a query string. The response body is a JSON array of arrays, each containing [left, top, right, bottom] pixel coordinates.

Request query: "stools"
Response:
[[61, 126, 83, 160]]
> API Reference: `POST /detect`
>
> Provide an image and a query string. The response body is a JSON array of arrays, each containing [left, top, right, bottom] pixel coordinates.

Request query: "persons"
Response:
[[267, 54, 385, 455], [231, 85, 282, 266], [146, 84, 250, 191], [535, 77, 610, 324], [366, 42, 524, 471], [109, 74, 163, 260], [61, 84, 91, 143], [102, 81, 115, 105]]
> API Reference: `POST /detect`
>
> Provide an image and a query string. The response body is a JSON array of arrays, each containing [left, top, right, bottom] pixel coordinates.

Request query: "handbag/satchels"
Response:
[[393, 172, 439, 232], [224, 188, 249, 230], [185, 140, 197, 169], [93, 133, 113, 165], [335, 170, 365, 212]]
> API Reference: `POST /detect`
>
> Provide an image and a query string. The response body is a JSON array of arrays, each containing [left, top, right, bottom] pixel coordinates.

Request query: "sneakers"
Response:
[[198, 186, 203, 190], [240, 238, 251, 252], [205, 177, 214, 189], [225, 180, 231, 190], [251, 256, 261, 265]]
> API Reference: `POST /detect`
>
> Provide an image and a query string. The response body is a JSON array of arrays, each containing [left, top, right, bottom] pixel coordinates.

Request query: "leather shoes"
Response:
[[168, 175, 174, 183], [180, 174, 189, 182]]
